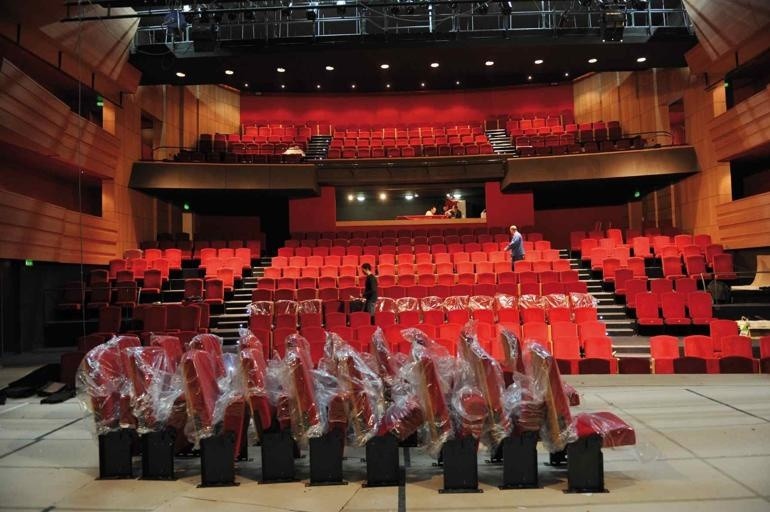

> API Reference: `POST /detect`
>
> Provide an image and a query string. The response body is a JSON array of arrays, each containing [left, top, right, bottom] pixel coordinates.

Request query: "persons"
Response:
[[452, 205, 461, 218], [426, 207, 437, 217], [443, 207, 452, 218], [504, 226, 524, 271], [362, 263, 378, 313]]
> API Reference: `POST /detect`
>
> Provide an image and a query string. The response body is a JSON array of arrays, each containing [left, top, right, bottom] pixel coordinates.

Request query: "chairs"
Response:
[[56, 238, 261, 371], [205, 112, 645, 162], [570, 227, 770, 374], [242, 225, 617, 374], [84, 328, 638, 494]]
